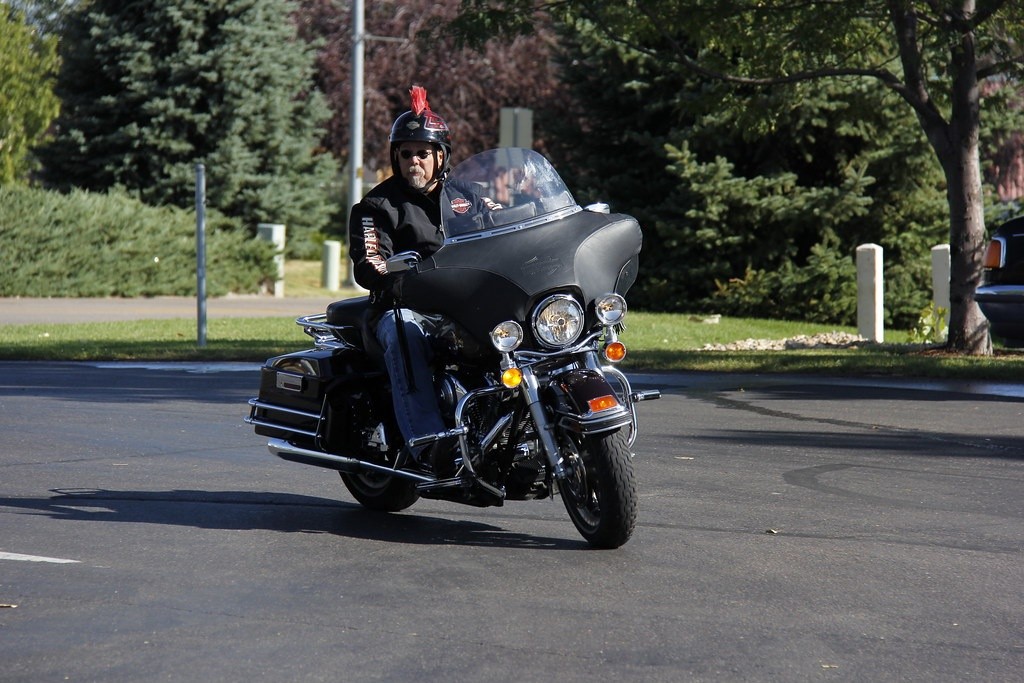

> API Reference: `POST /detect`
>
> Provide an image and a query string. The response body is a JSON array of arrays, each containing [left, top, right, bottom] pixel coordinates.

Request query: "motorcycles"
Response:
[[244, 146, 662, 550]]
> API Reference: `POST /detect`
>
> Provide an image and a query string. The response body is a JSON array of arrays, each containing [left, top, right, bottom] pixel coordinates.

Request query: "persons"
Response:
[[349, 84, 512, 479]]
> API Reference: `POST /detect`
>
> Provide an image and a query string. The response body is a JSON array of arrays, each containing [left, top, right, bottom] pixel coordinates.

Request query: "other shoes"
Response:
[[415, 446, 463, 475]]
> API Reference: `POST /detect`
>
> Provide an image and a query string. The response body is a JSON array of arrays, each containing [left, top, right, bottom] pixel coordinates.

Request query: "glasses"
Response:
[[394, 149, 434, 159]]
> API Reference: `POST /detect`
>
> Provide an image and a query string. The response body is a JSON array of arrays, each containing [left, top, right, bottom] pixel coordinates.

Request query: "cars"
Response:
[[974, 215, 1024, 356]]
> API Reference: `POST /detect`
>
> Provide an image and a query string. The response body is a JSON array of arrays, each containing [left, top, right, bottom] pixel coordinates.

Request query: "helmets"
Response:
[[388, 85, 452, 148]]
